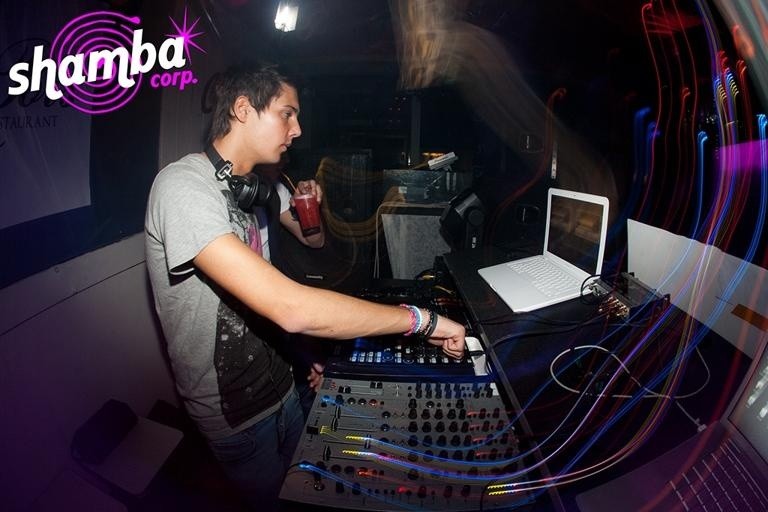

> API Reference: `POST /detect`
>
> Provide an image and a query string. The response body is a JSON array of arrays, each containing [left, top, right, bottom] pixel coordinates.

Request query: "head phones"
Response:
[[202, 142, 277, 211]]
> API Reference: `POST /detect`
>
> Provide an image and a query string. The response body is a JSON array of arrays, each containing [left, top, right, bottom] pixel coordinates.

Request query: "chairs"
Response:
[[70, 398, 182, 495]]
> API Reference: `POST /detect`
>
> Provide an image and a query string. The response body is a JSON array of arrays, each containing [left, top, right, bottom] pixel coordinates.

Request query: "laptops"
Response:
[[575, 329, 768, 512], [477, 188, 610, 315]]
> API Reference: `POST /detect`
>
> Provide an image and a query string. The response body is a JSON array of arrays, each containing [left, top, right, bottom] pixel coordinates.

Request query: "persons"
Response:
[[144, 63, 466, 512]]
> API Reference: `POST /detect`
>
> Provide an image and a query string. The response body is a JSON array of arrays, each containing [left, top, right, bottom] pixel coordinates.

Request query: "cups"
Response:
[[292, 193, 322, 231]]
